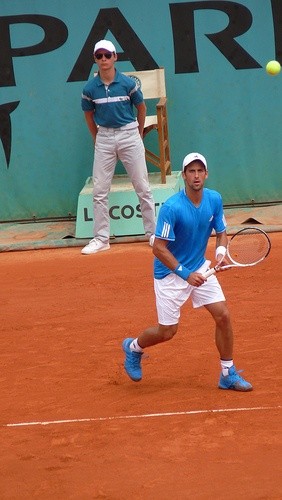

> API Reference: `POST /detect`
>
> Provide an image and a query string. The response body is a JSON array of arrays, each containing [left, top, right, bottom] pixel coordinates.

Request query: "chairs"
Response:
[[93, 67, 171, 184]]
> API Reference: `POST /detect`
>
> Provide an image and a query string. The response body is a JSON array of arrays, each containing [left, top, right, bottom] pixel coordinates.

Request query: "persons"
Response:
[[122, 153, 253, 392], [80, 40, 156, 255]]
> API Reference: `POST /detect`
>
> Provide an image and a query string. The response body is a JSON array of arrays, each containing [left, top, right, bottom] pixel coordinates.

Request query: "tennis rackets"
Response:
[[201, 228, 271, 279]]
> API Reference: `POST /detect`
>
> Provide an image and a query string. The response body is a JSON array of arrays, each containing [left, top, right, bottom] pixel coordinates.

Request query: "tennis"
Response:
[[265, 60, 281, 76]]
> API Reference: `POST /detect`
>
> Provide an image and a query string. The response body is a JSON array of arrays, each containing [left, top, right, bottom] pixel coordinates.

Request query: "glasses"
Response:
[[96, 53, 111, 59]]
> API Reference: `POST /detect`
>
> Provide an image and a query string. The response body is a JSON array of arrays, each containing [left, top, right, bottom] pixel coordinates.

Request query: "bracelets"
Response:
[[215, 246, 226, 260], [173, 263, 192, 280]]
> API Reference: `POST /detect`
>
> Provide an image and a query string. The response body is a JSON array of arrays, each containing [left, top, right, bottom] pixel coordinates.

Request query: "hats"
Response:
[[183, 153, 207, 172], [94, 40, 116, 54]]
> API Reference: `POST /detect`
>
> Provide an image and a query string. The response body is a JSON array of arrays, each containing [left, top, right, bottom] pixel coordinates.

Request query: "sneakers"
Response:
[[218, 365, 253, 391], [122, 338, 144, 382], [149, 234, 155, 247], [80, 238, 111, 255]]
